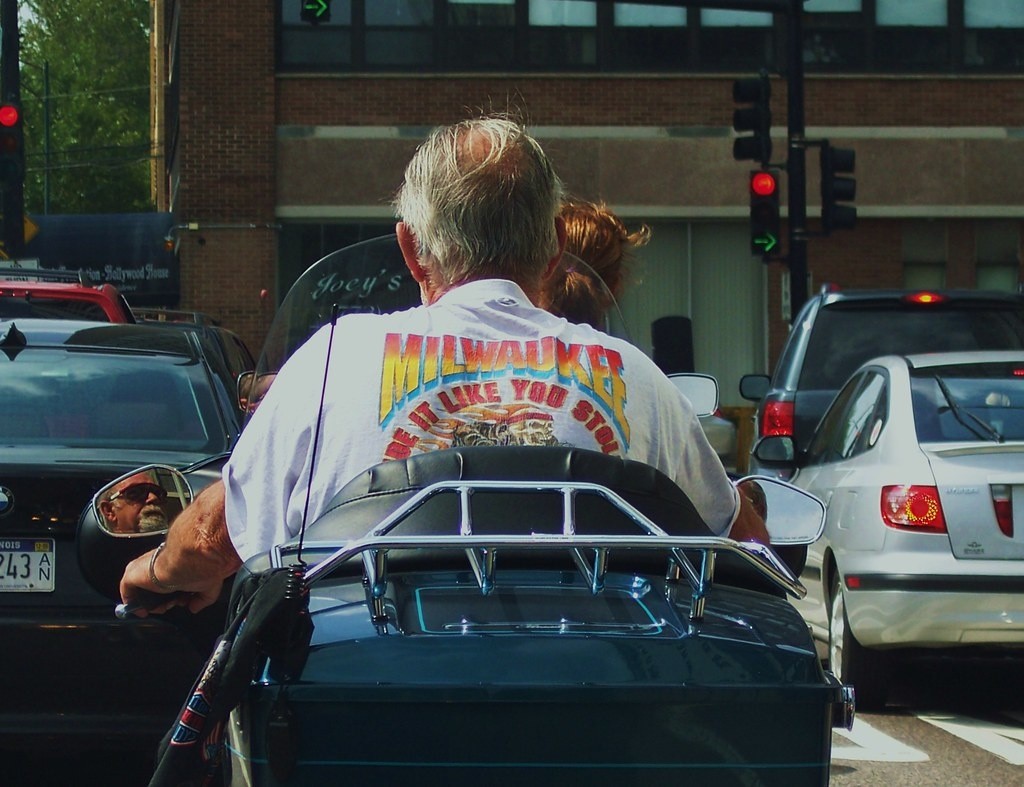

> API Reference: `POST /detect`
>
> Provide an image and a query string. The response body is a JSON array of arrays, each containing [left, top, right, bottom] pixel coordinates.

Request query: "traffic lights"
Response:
[[0, 89, 27, 196], [747, 168, 784, 259], [820, 137, 859, 232], [301, 0, 332, 23], [731, 72, 773, 164]]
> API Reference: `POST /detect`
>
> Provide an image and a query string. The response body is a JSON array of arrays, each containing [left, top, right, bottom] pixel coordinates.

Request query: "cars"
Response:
[[751, 350, 1024, 711]]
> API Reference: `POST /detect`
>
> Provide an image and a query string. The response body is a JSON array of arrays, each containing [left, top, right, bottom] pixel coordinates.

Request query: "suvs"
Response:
[[737, 281, 1024, 582], [1, 265, 137, 325], [0, 313, 278, 787]]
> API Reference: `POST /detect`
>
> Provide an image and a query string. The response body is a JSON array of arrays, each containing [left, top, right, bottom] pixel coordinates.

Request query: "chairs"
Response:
[[911, 389, 944, 442], [0, 400, 50, 437], [69, 402, 179, 439]]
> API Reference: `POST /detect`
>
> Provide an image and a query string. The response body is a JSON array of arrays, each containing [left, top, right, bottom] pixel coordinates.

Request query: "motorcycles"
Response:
[[90, 227, 856, 787]]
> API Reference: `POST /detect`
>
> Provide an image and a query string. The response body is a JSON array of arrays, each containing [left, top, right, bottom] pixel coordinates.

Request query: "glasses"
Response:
[[97, 483, 168, 509]]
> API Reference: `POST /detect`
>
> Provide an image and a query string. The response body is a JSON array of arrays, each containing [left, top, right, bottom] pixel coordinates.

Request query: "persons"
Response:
[[537, 195, 651, 331], [97, 472, 167, 534], [120, 119, 770, 618]]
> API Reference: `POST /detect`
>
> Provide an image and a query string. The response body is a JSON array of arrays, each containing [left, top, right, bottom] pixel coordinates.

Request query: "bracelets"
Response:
[[150, 541, 189, 589]]
